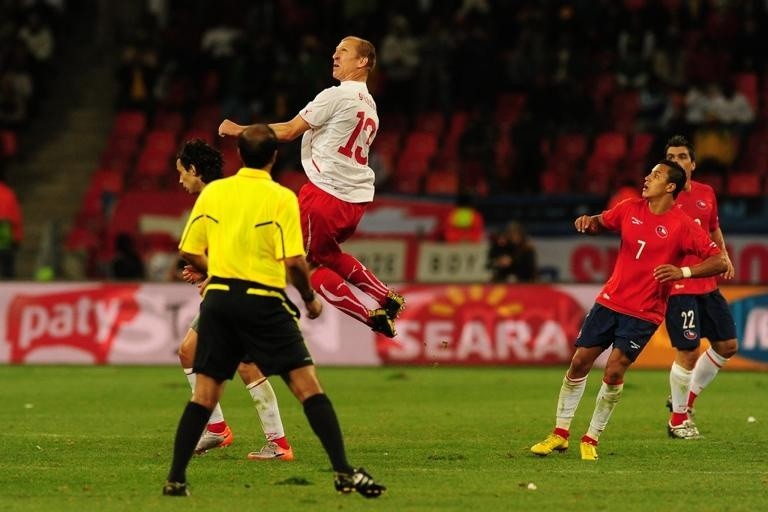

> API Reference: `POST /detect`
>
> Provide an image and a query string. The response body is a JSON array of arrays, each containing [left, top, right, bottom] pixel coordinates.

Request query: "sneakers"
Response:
[[247, 440, 293, 461], [579, 440, 598, 460], [163, 480, 191, 495], [334, 468, 386, 498], [530, 431, 569, 456], [666, 395, 702, 439], [368, 291, 406, 338], [194, 425, 232, 454]]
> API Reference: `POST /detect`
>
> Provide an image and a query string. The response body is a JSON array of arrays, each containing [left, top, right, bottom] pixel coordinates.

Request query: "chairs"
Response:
[[63, 98, 270, 254], [377, 68, 765, 214]]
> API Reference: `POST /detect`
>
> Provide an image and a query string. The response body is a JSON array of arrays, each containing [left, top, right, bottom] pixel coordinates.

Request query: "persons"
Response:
[[218, 37, 405, 339], [161, 121, 389, 500], [660, 132, 740, 442], [531, 160, 729, 463], [172, 135, 297, 462], [0, 0, 768, 290]]
